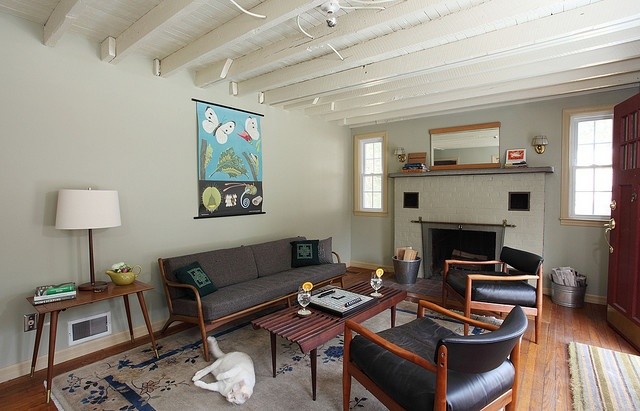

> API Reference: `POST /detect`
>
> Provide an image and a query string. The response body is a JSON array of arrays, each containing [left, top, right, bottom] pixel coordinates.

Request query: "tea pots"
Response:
[[106, 263, 141, 286]]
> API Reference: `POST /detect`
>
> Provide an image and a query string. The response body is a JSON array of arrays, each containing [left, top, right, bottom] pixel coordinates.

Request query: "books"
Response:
[[400, 162, 428, 172], [552, 266, 586, 287], [32, 282, 77, 306]]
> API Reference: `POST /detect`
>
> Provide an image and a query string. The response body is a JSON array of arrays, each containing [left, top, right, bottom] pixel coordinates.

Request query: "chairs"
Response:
[[440, 246, 545, 344], [340, 300, 527, 408]]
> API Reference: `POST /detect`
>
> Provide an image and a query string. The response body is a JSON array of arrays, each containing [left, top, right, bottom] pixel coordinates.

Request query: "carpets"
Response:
[[59, 301, 507, 410], [567, 341, 640, 411]]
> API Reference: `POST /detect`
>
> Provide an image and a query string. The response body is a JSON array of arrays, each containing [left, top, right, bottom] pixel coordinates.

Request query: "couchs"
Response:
[[156, 237, 346, 361]]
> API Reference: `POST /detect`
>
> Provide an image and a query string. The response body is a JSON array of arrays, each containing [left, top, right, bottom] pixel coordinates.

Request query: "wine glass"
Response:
[[297, 285, 312, 315], [369, 276, 384, 297]]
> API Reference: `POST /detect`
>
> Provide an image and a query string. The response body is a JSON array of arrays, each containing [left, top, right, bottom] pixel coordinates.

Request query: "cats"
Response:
[[192, 336, 255, 405]]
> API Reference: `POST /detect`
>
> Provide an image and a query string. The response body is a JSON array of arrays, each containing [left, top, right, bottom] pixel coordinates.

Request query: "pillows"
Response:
[[173, 261, 218, 300], [289, 239, 322, 268]]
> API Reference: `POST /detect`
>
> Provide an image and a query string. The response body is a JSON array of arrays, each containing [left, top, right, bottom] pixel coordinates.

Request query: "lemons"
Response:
[[376, 268, 384, 277], [303, 282, 313, 292]]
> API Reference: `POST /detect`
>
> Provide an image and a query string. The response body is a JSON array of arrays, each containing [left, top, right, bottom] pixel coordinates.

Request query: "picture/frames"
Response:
[[504, 148, 527, 164]]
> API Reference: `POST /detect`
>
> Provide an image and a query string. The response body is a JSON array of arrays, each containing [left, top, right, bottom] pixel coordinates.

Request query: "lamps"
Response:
[[393, 147, 407, 163], [53, 188, 121, 291], [533, 135, 549, 154]]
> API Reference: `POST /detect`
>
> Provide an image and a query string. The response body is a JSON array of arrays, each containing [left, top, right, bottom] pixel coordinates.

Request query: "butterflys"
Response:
[[243, 116, 260, 143], [201, 105, 236, 145]]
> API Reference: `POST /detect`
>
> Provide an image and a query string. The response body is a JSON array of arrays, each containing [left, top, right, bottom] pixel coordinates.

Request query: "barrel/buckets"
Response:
[[391, 254, 422, 285], [549, 279, 589, 309]]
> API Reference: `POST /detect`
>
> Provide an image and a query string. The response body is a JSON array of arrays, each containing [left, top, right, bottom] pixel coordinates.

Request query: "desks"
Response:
[[25, 278, 161, 404]]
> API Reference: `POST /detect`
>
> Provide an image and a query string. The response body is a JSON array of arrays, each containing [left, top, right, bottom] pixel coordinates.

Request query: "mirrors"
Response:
[[429, 122, 502, 168]]
